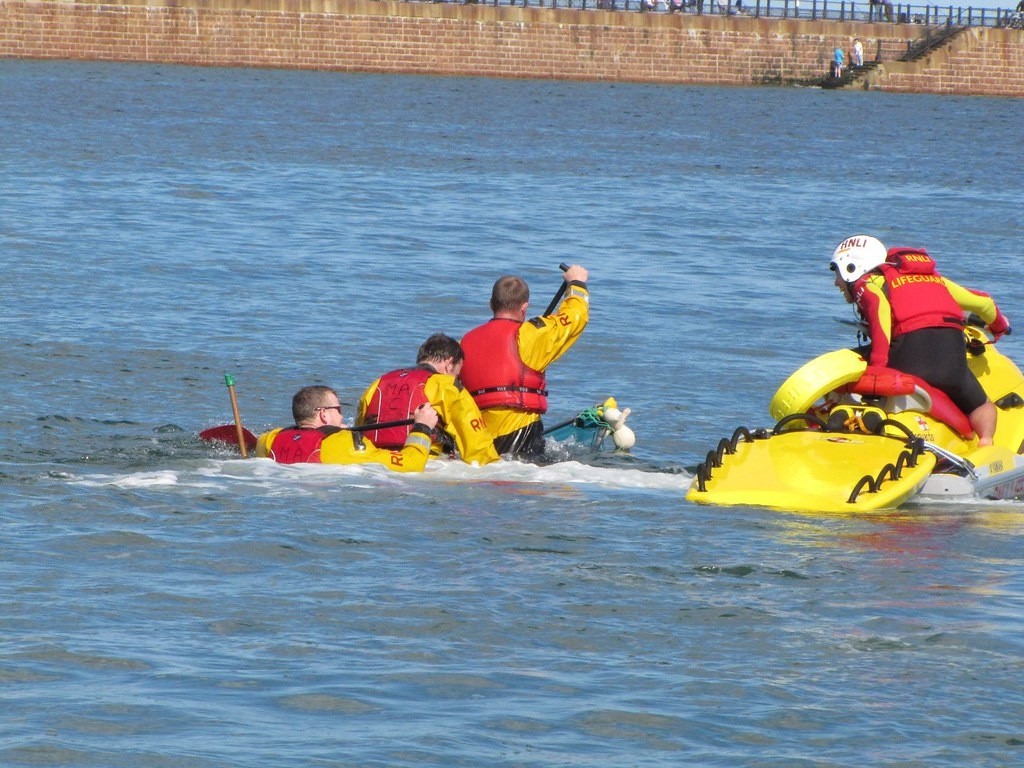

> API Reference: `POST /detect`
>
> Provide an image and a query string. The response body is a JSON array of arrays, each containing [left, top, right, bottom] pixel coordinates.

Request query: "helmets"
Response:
[[830, 236, 887, 285]]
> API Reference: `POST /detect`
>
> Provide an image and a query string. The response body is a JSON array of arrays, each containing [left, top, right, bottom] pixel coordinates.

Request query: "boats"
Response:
[[544, 399, 624, 453]]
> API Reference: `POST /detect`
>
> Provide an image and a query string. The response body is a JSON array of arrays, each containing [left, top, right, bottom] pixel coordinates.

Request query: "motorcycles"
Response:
[[687, 317, 1024, 508]]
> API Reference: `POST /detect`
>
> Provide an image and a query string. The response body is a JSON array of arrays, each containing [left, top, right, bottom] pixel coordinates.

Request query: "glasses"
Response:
[[315, 406, 342, 416]]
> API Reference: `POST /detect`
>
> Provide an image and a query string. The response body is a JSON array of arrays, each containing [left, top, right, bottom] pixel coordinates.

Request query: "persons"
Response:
[[825, 234, 1014, 450], [832, 46, 844, 78], [1016, 0, 1024, 25], [256, 385, 439, 476], [853, 38, 864, 66], [457, 262, 592, 460], [351, 331, 504, 467]]
